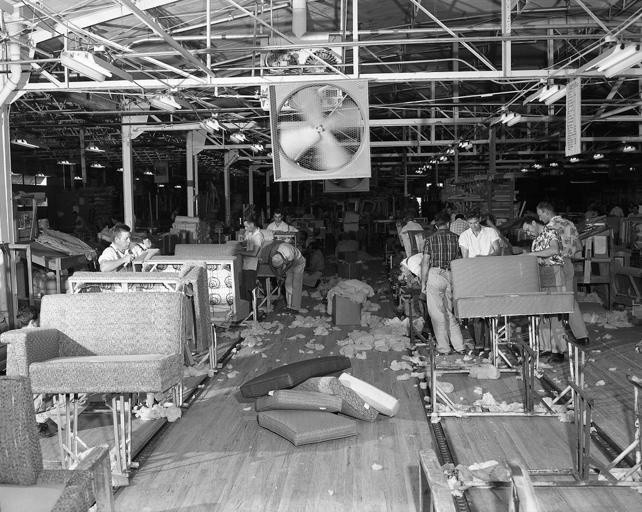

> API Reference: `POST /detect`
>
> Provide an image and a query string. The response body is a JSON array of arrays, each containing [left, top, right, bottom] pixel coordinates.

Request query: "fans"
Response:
[[268, 36, 373, 194]]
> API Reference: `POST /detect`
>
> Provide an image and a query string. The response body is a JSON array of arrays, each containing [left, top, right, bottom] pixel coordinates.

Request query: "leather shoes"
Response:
[[548, 354, 564, 365], [540, 350, 551, 356], [579, 338, 589, 345]]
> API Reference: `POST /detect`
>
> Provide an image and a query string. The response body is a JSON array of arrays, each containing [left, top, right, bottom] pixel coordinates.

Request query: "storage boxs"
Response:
[[331, 293, 361, 326]]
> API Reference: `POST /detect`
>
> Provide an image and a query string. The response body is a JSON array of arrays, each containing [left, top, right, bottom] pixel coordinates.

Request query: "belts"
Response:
[[433, 266, 450, 270]]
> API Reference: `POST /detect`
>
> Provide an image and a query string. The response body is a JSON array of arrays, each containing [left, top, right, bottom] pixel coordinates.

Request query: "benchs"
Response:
[[450, 256, 575, 372]]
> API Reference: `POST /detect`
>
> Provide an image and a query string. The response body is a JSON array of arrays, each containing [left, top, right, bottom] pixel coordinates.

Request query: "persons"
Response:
[[397, 202, 641, 364], [52, 203, 187, 291], [232, 208, 325, 322]]
